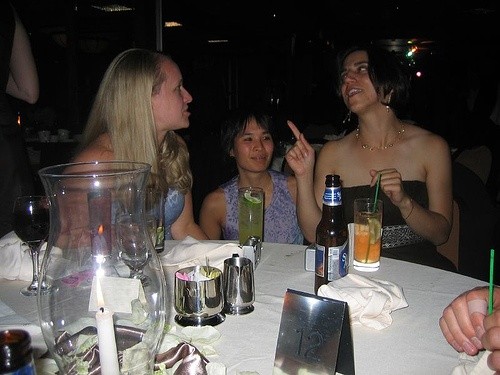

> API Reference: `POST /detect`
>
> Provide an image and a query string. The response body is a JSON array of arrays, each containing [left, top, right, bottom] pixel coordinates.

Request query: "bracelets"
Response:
[[402, 197, 414, 219]]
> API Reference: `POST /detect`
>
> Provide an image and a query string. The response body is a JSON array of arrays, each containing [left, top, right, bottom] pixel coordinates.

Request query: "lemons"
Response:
[[242, 189, 262, 206], [366, 217, 381, 244]]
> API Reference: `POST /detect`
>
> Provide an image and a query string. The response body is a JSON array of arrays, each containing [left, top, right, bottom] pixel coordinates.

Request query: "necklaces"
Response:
[[355, 118, 406, 150]]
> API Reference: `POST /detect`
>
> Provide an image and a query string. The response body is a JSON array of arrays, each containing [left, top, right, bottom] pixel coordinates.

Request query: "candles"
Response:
[[95, 274, 119, 375]]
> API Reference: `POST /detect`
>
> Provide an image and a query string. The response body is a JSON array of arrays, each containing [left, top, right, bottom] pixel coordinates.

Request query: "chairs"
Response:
[[449, 143, 495, 256]]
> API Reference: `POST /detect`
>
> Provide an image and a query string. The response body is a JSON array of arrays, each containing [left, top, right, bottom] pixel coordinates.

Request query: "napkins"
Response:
[[317, 274, 409, 330], [0, 301, 42, 335], [160, 235, 241, 273], [0, 231, 63, 281]]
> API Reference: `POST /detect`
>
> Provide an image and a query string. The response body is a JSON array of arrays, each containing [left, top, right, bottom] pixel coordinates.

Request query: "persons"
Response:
[[285, 45, 458, 272], [439, 286, 500, 375], [198, 104, 305, 245], [56, 48, 207, 250], [0, 0, 40, 237]]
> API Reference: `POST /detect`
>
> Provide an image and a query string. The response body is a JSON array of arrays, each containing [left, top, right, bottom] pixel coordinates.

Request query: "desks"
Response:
[[0, 240, 500, 375]]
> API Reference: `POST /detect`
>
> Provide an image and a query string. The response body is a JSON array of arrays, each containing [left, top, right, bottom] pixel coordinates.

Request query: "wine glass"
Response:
[[14, 195, 59, 296], [113, 212, 152, 287]]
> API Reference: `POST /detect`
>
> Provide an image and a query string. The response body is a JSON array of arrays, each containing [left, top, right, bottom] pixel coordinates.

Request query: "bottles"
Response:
[[313, 175, 349, 296], [0, 329, 37, 375], [37, 161, 167, 375]]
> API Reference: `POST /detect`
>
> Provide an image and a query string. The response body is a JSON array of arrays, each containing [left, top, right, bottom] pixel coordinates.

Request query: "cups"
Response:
[[223, 253, 255, 316], [237, 186, 263, 246], [174, 266, 226, 326], [144, 187, 165, 253], [352, 198, 384, 272]]
